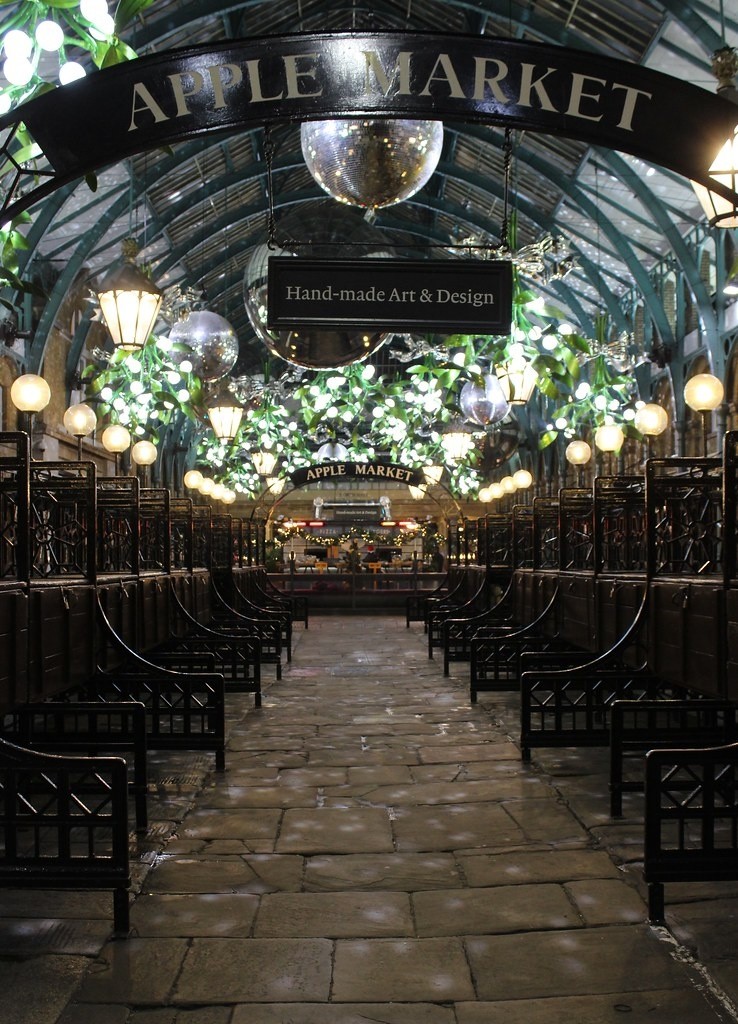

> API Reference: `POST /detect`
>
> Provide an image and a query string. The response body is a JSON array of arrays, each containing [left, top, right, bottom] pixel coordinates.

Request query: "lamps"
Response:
[[408, 482, 427, 500], [100, 155, 160, 353], [184, 469, 236, 504], [11, 374, 158, 489], [494, 352, 538, 404], [423, 462, 444, 487], [691, 0, 738, 227], [266, 477, 286, 496], [207, 378, 245, 445], [441, 412, 474, 460], [566, 373, 725, 488], [479, 470, 533, 502], [250, 443, 278, 478]]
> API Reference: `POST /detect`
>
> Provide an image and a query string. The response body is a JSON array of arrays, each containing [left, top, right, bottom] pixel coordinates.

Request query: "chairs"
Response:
[[300, 562, 425, 588]]
